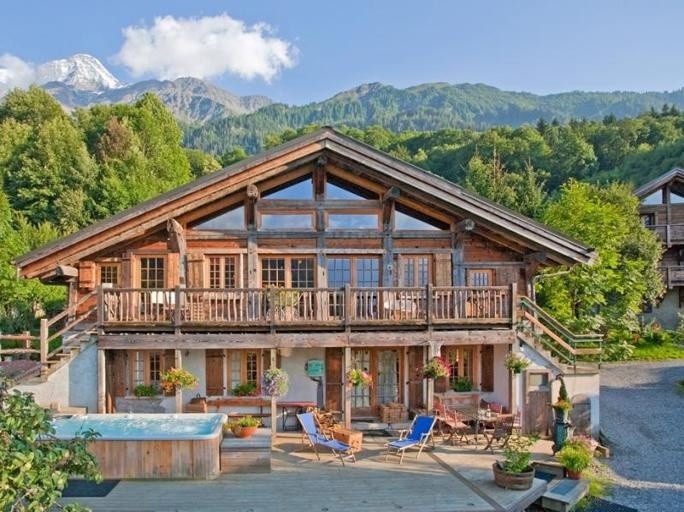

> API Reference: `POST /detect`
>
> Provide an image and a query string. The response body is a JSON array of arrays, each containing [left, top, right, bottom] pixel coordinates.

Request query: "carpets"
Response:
[[54, 479, 121, 497]]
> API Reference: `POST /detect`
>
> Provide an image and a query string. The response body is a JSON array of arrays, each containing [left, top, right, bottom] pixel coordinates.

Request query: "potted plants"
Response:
[[263, 284, 303, 323], [231, 383, 256, 397], [223, 414, 263, 438], [503, 349, 531, 374], [492, 432, 541, 490], [554, 444, 592, 480]]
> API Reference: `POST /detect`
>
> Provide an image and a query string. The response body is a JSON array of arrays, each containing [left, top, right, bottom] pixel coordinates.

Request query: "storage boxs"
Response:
[[333, 429, 363, 455], [377, 401, 409, 424]]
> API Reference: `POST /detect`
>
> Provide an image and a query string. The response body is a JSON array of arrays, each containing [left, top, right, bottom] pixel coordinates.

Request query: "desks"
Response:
[[276, 401, 319, 431], [207, 399, 271, 417]]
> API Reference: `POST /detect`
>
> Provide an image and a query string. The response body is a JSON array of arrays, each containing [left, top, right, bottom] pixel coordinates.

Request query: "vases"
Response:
[[353, 381, 360, 386], [553, 408, 568, 423], [426, 371, 432, 378], [164, 386, 176, 397]]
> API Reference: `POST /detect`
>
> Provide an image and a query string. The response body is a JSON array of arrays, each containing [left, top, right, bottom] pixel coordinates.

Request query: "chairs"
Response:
[[101, 282, 118, 320], [384, 402, 522, 464], [382, 290, 416, 319], [296, 412, 356, 467], [151, 289, 190, 323]]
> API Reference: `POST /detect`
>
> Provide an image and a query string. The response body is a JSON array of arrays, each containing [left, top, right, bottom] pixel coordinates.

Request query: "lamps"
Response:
[[387, 260, 393, 271]]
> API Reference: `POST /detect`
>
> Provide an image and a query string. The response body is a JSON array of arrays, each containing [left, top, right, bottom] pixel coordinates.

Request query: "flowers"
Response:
[[545, 398, 573, 407], [417, 358, 452, 378], [259, 368, 289, 397], [158, 366, 199, 390], [345, 367, 373, 384]]
